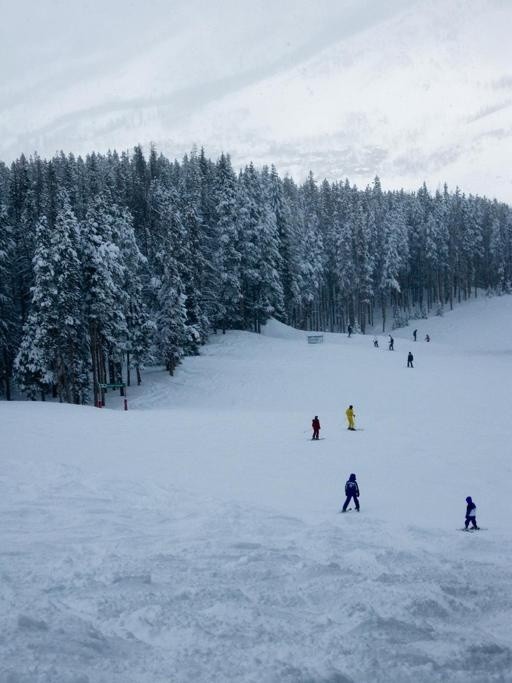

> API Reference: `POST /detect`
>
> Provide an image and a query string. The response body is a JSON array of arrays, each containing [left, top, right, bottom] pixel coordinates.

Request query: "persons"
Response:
[[462, 495, 479, 529], [412, 327, 418, 341], [347, 321, 353, 337], [388, 334, 394, 351], [345, 404, 355, 430], [342, 472, 360, 512], [406, 350, 414, 368], [310, 415, 321, 441], [423, 334, 429, 342]]
